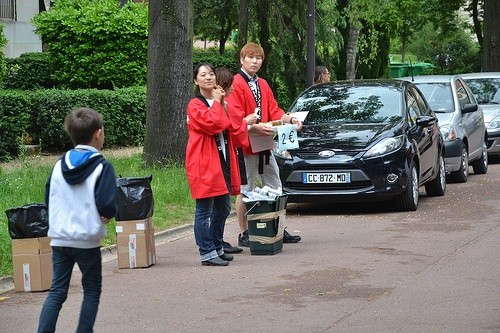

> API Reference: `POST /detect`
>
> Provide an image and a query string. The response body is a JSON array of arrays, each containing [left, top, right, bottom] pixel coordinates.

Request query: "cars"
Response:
[[271, 78, 446, 211], [453, 72, 500, 160], [360, 74, 488, 184]]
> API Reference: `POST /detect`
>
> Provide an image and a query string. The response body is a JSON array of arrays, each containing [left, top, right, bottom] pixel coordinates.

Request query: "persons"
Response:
[[185, 41, 304, 267], [36, 108, 119, 332], [315, 66, 331, 85]]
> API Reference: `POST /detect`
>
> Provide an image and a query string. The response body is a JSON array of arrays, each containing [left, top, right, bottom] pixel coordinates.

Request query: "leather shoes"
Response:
[[202, 255, 229, 266], [220, 254, 234, 261]]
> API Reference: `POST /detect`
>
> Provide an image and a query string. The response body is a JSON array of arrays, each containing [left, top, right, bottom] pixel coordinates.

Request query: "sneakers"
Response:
[[284, 230, 301, 242], [238, 230, 250, 247]]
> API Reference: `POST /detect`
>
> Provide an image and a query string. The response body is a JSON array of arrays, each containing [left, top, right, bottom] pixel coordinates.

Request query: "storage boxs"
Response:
[[10, 237, 54, 292], [243, 185, 287, 256], [115, 217, 157, 269], [248, 120, 299, 155]]
